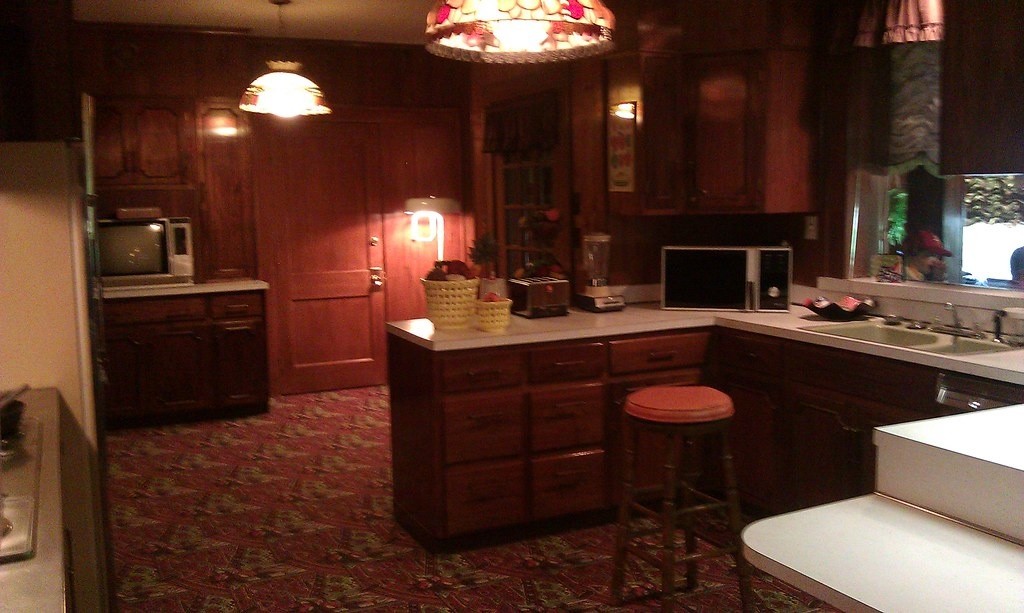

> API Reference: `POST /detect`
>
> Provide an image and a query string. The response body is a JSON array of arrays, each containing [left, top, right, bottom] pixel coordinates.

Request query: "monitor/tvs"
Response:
[[97, 214, 194, 292]]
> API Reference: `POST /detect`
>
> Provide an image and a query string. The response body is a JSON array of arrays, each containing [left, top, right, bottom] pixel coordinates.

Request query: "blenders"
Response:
[[573, 233, 627, 312]]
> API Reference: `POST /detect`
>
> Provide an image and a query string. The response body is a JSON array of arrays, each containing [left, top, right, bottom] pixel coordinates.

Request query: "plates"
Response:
[[805, 299, 878, 318]]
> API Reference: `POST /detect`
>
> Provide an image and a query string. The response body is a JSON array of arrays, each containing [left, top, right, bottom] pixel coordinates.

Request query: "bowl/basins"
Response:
[[1, 400, 24, 443]]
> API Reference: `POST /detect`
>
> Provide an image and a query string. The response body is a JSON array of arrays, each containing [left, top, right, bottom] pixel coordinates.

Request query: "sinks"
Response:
[[816, 323, 938, 347], [922, 340, 1007, 355]]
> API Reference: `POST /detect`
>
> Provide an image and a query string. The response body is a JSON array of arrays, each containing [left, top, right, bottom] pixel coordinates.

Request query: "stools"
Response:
[[611, 383, 756, 613]]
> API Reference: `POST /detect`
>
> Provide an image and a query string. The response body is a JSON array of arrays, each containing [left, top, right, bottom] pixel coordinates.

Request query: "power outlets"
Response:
[[804, 213, 818, 240]]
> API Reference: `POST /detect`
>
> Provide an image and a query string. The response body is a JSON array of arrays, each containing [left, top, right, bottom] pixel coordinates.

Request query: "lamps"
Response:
[[239, 0, 333, 117], [423, 0, 617, 65]]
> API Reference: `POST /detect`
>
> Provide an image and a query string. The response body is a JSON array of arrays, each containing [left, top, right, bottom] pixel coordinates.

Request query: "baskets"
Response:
[[420, 278, 479, 331], [478, 299, 512, 333]]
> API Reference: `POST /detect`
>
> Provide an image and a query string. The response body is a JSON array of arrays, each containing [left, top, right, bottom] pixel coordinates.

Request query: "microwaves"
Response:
[[660, 244, 794, 313]]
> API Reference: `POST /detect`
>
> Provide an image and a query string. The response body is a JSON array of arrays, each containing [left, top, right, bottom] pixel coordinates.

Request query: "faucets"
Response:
[[943, 302, 962, 332]]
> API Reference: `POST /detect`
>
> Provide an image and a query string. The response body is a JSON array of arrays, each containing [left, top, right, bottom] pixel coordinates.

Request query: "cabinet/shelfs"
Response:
[[784, 339, 936, 515], [92, 97, 202, 193], [610, 328, 719, 510], [713, 324, 788, 522], [691, 49, 824, 215], [103, 292, 216, 425], [605, 50, 688, 217], [211, 290, 269, 414], [386, 334, 613, 549]]
